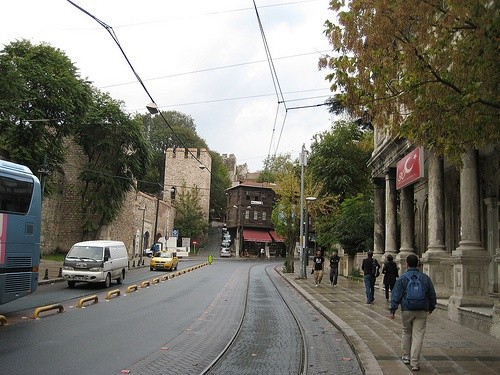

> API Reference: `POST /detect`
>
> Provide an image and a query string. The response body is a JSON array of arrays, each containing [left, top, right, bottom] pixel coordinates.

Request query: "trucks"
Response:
[[143, 236, 191, 259]]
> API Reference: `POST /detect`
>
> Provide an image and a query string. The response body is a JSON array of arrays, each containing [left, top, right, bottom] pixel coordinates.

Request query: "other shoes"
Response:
[[411, 364, 421, 370], [402, 357, 410, 363]]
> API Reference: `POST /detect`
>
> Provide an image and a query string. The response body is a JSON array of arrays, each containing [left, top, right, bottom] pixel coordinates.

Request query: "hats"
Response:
[[367, 252, 373, 255]]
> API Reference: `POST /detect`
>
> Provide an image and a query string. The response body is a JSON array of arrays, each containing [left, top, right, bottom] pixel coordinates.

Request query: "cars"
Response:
[[221, 223, 232, 247], [149, 249, 179, 272], [219, 247, 232, 257]]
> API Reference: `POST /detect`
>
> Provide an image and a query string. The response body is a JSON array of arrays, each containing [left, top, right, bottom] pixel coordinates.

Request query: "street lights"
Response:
[[304, 196, 317, 279]]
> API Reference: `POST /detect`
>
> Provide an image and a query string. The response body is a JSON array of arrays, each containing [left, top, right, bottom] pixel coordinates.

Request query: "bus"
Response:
[[0, 159, 42, 305]]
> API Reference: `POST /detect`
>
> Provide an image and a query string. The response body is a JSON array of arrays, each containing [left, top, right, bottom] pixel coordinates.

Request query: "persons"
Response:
[[382, 255, 399, 301], [389, 255, 437, 370], [329, 250, 340, 288], [312, 250, 324, 287], [361, 251, 380, 303]]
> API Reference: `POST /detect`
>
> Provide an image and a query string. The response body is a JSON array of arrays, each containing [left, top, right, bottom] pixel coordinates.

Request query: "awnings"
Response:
[[270, 231, 285, 242], [243, 229, 272, 244]]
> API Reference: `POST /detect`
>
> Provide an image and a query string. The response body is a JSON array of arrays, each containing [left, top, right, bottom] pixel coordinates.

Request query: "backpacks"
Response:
[[403, 272, 424, 303], [365, 258, 380, 278]]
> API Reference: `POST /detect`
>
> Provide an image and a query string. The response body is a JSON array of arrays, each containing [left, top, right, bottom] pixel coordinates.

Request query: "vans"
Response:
[[61, 239, 129, 289]]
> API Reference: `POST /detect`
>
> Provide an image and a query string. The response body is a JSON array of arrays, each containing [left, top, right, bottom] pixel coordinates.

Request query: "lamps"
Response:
[[199, 164, 205, 170], [146, 103, 158, 114]]
